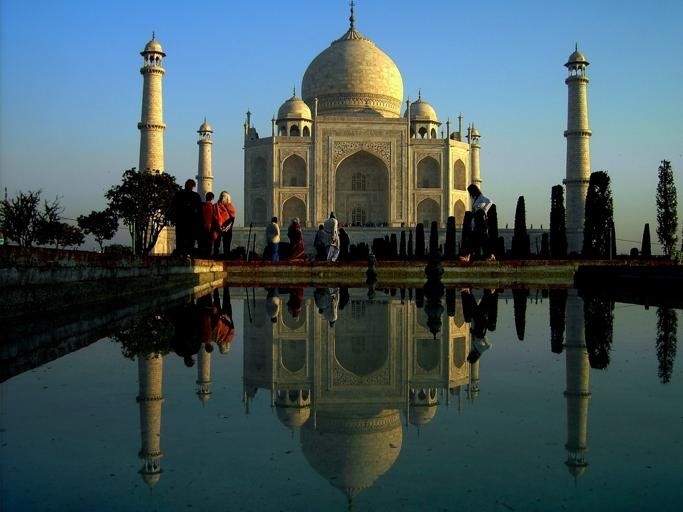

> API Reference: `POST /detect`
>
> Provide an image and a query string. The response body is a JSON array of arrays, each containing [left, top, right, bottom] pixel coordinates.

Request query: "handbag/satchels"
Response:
[[219, 216, 234, 235]]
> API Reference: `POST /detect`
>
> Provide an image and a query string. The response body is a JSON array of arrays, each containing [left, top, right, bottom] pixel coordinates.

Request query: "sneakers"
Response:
[[489, 254, 496, 260], [458, 254, 471, 262]]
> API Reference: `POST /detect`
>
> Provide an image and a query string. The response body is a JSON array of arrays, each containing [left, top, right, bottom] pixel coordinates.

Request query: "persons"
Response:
[[321, 212, 340, 262], [286, 288, 303, 322], [212, 191, 235, 259], [287, 217, 304, 259], [458, 184, 496, 262], [196, 191, 214, 256], [313, 224, 324, 259], [170, 179, 204, 256], [166, 298, 201, 368], [338, 288, 349, 310], [456, 288, 496, 363], [264, 287, 281, 323], [211, 284, 235, 355], [196, 293, 217, 354], [265, 217, 280, 261], [337, 228, 349, 259], [322, 288, 340, 327], [313, 287, 330, 313]]
